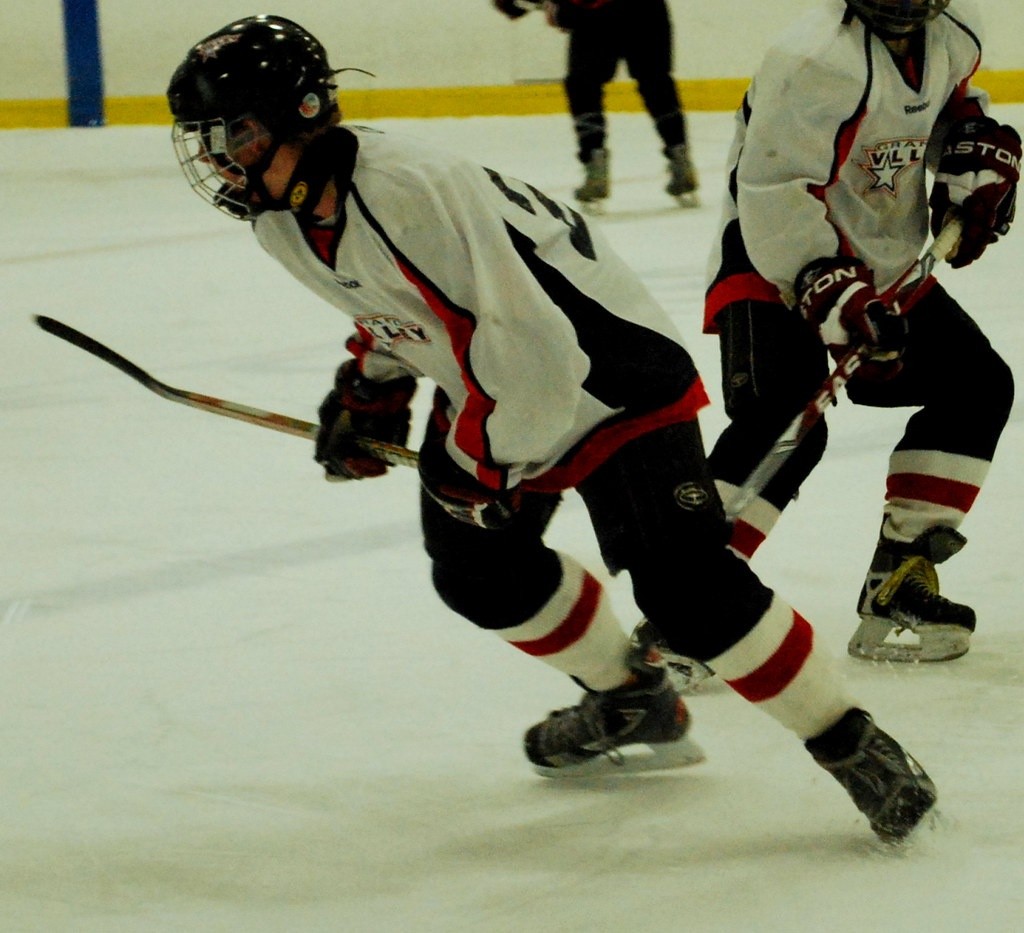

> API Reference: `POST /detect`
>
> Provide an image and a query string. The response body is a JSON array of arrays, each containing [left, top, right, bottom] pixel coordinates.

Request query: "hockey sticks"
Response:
[[29, 312, 508, 517], [631, 185, 1001, 656]]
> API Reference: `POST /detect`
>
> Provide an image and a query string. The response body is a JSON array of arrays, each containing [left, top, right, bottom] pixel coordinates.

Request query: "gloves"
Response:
[[928, 117, 1021, 268], [314, 358, 417, 484], [792, 256, 908, 385], [414, 433, 514, 529]]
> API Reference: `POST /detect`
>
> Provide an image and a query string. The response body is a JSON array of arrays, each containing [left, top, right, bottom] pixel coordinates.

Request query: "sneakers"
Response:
[[629, 620, 715, 689], [804, 707, 946, 859], [525, 660, 706, 778], [664, 144, 703, 210], [847, 512, 976, 664], [574, 149, 609, 216]]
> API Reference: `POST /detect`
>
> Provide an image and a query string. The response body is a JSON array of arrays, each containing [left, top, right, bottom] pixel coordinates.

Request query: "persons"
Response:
[[489, 1, 702, 211], [165, 14, 945, 849], [625, 1, 1023, 694]]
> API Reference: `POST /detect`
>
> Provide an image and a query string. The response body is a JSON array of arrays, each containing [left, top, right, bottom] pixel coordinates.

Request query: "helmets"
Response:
[[167, 15, 342, 226], [843, 0, 950, 38]]
